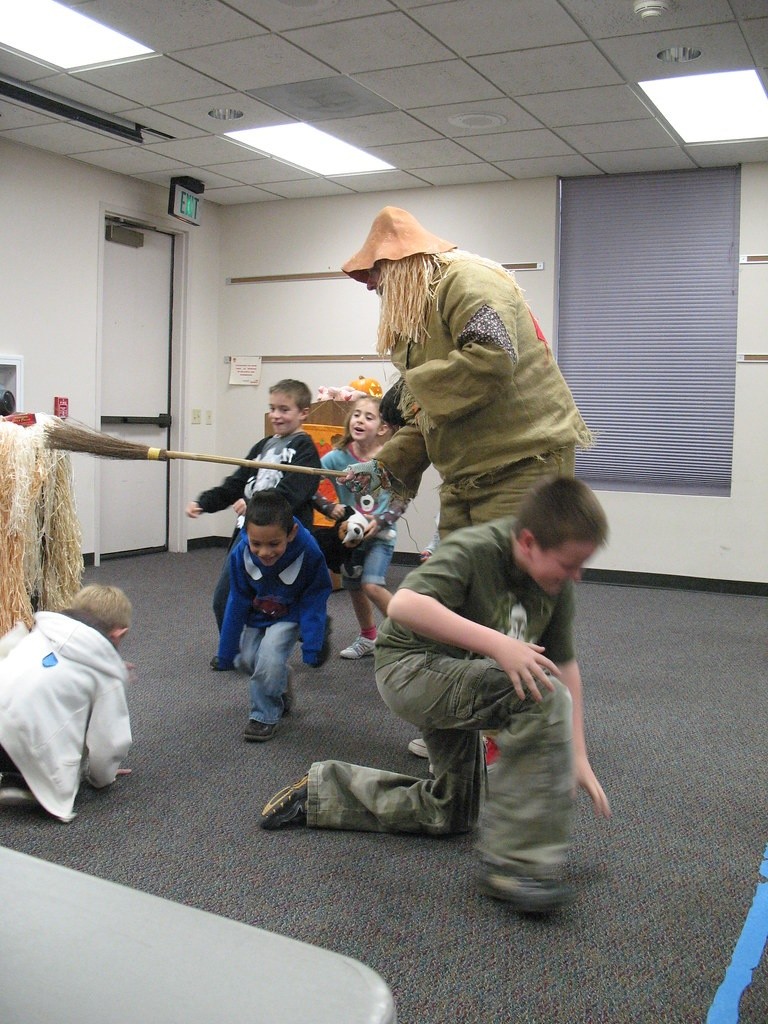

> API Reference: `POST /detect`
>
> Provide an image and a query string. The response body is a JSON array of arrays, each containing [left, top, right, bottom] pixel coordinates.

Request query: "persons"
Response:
[[186, 379, 333, 670], [310, 396, 393, 659], [338, 206, 593, 756], [212, 487, 332, 743], [0, 585, 133, 823], [256, 476, 613, 911]]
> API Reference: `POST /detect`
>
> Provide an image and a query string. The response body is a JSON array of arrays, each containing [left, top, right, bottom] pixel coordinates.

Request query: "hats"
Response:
[[341, 206, 458, 283]]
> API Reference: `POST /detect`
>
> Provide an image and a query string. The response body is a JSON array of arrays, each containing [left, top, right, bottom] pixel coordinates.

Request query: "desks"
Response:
[[0, 848, 397, 1024]]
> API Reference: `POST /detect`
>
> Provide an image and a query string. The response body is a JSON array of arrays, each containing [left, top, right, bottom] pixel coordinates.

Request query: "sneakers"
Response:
[[340, 636, 379, 659], [478, 863, 579, 910], [260, 772, 308, 831]]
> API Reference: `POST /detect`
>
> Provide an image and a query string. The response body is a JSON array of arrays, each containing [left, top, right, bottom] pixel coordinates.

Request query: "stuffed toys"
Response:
[[312, 504, 370, 574], [316, 386, 367, 401]]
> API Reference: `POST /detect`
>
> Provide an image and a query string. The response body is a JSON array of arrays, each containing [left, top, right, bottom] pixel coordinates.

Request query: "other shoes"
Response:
[[283, 664, 294, 714], [1, 773, 37, 807], [244, 719, 279, 741], [409, 738, 429, 758]]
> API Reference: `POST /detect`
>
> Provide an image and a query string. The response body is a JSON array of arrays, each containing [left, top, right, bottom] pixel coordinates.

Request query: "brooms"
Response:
[[39, 413, 372, 484]]
